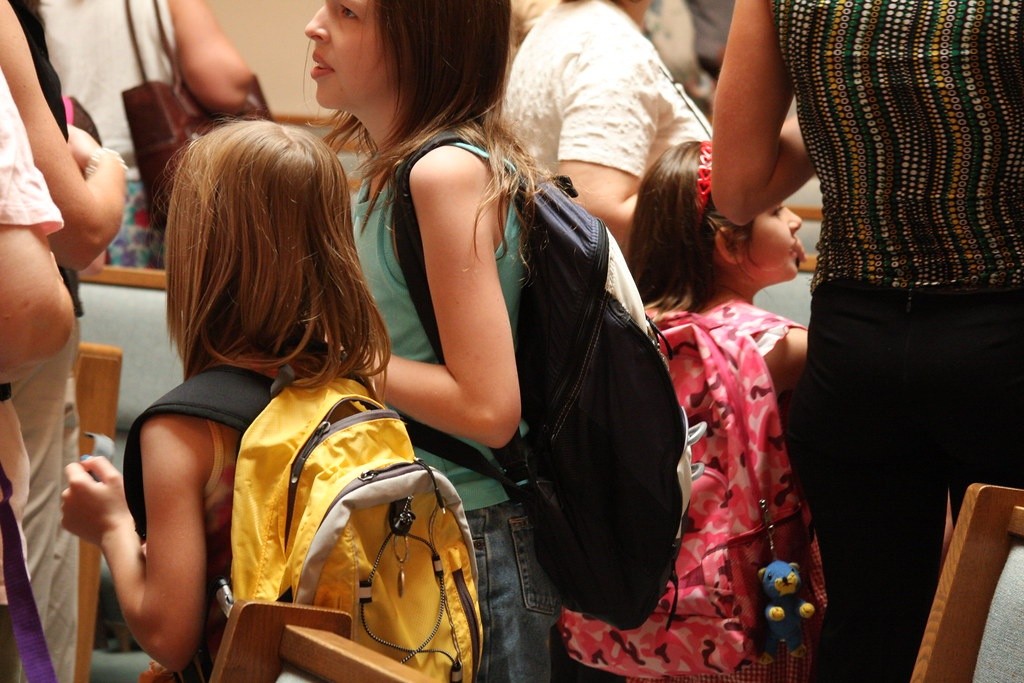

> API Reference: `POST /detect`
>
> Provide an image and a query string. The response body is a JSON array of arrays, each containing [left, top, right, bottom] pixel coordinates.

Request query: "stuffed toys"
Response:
[[756, 561, 816, 663]]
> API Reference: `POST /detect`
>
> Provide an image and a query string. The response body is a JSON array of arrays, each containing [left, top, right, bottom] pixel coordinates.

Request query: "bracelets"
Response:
[[84, 147, 128, 180]]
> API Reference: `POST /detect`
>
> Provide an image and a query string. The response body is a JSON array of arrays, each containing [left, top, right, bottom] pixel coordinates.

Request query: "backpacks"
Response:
[[123, 363, 484, 683], [394, 127, 708, 630], [557, 311, 827, 680]]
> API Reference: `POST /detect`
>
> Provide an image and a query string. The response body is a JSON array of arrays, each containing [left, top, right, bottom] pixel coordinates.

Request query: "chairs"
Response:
[[66, 172, 1024, 683]]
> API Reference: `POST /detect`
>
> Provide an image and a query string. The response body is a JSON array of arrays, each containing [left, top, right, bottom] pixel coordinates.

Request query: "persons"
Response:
[[556, 140, 829, 681], [62, 117, 392, 683], [713, 1, 1024, 682], [299, 1, 560, 682], [487, 0, 730, 283], [0, 2, 270, 683]]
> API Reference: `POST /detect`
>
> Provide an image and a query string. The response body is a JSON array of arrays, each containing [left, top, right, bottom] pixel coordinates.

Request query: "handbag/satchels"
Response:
[[122, 0, 274, 228]]
